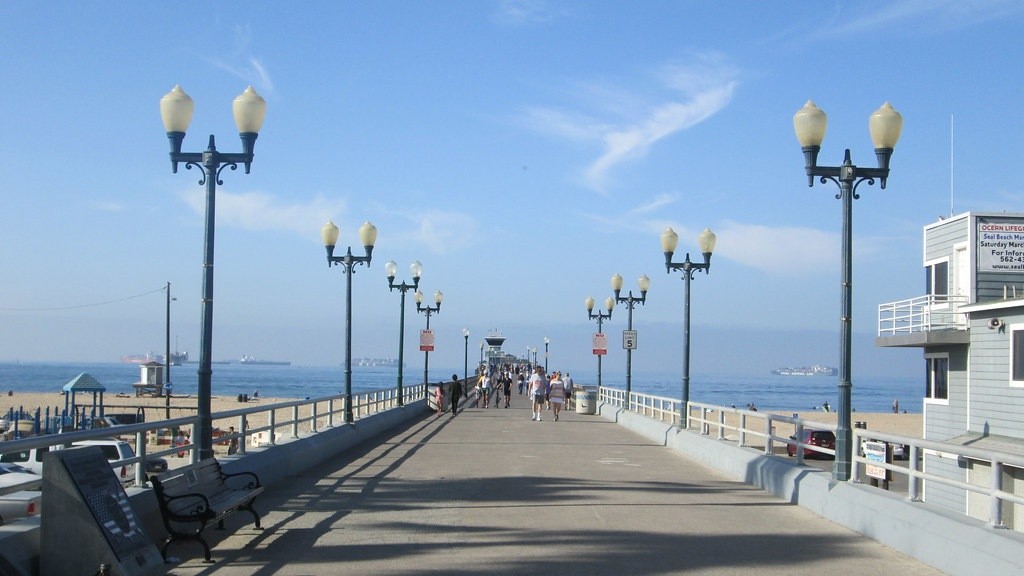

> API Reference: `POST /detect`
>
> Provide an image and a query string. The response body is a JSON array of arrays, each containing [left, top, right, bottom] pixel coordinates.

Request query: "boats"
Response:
[[770, 364, 838, 376], [352, 358, 397, 368], [240, 354, 291, 365], [121, 352, 189, 366]]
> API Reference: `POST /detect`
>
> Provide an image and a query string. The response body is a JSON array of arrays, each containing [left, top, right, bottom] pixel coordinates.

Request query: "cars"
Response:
[[0, 411, 168, 525], [786, 428, 904, 460]]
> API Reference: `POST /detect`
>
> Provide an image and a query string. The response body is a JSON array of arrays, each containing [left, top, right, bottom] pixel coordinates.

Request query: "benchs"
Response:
[[150, 457, 267, 564]]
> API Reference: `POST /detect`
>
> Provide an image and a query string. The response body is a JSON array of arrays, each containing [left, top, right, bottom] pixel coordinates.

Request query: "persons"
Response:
[[550, 370, 565, 421], [822, 401, 829, 412], [434, 382, 446, 417], [545, 374, 552, 410], [528, 365, 545, 421], [517, 373, 525, 395], [497, 362, 532, 388], [228, 427, 239, 455], [477, 365, 493, 408], [448, 374, 462, 415], [254, 391, 258, 398], [893, 400, 898, 413], [170, 424, 190, 458], [524, 371, 531, 395], [496, 372, 512, 409], [562, 372, 573, 410]]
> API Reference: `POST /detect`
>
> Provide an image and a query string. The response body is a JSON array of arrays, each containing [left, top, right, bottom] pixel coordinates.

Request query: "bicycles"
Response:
[[492, 388, 503, 409], [474, 386, 481, 408]]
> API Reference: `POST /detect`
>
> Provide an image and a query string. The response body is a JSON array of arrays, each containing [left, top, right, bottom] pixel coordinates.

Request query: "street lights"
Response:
[[585, 272, 650, 410], [160, 84, 267, 458], [321, 218, 377, 422], [384, 260, 443, 406], [463, 328, 497, 397], [660, 227, 717, 429], [522, 337, 550, 374], [792, 99, 903, 481]]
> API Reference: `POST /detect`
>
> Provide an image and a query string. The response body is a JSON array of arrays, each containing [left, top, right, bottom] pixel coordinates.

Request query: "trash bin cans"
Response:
[[576, 391, 596, 415], [237, 394, 247, 401], [429, 388, 448, 411]]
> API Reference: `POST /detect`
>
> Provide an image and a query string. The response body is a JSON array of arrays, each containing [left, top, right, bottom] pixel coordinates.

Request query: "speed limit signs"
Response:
[[623, 330, 637, 348]]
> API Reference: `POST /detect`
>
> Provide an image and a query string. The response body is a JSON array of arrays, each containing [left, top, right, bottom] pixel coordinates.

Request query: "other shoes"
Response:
[[554, 418, 557, 421], [507, 403, 510, 407], [536, 417, 542, 421], [483, 404, 485, 408], [505, 405, 507, 408], [533, 415, 536, 420], [556, 415, 559, 421], [485, 405, 488, 409]]
